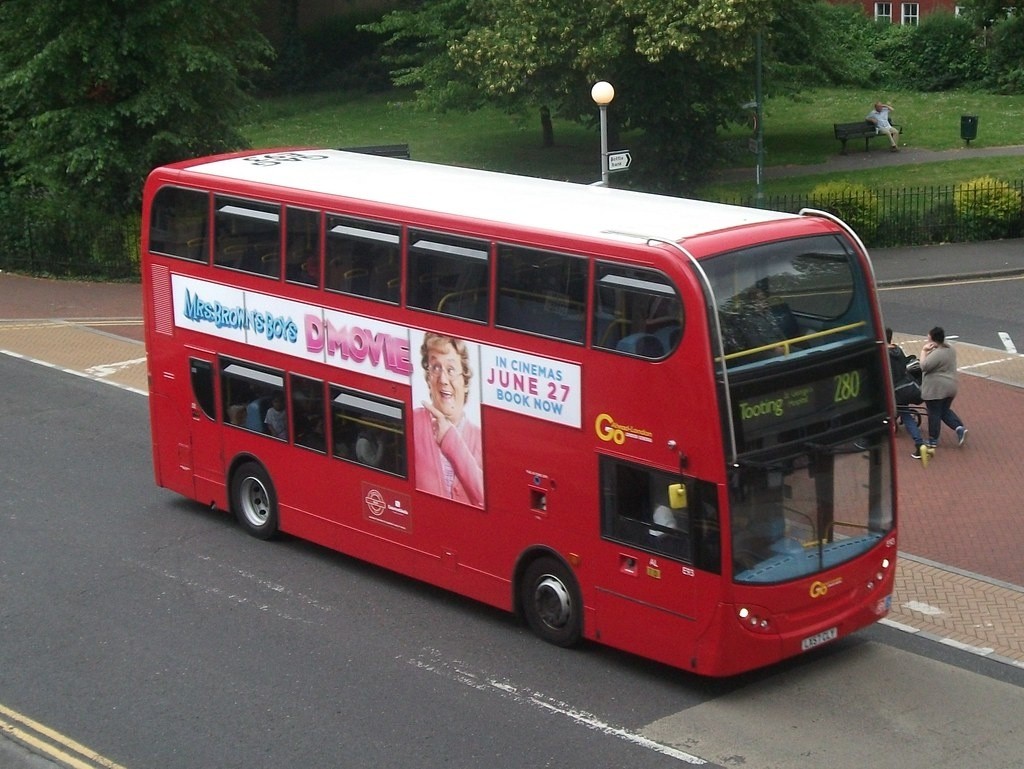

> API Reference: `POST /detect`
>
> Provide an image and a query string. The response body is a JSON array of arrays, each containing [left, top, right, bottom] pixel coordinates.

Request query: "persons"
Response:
[[731, 257, 802, 358], [231, 377, 260, 407], [699, 490, 770, 576], [886, 326, 928, 458], [229, 405, 249, 426], [865, 101, 900, 151], [263, 390, 288, 439], [305, 416, 327, 452], [307, 237, 332, 287], [293, 378, 316, 427], [412, 331, 484, 510], [920, 327, 968, 447], [347, 408, 393, 470]]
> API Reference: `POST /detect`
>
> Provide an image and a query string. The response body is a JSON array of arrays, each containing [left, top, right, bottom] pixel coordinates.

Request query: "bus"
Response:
[[140, 149, 899, 680]]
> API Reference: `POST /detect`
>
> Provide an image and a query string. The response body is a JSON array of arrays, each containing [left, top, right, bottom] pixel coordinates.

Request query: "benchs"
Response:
[[833, 116, 904, 156], [338, 143, 410, 161]]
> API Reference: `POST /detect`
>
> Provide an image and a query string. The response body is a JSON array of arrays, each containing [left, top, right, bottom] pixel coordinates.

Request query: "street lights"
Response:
[[591, 81, 615, 186]]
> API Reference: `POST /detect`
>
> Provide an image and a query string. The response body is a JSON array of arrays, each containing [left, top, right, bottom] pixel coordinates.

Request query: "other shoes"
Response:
[[911, 451, 921, 458], [926, 439, 938, 448], [957, 427, 969, 448], [891, 145, 899, 152], [854, 441, 867, 450]]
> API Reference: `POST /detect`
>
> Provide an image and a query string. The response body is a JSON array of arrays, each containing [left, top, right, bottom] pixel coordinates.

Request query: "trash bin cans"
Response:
[[961, 114, 979, 139]]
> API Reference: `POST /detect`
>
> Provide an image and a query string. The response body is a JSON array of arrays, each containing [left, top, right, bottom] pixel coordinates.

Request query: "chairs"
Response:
[[242, 397, 276, 436], [186, 236, 564, 317], [615, 325, 686, 362]]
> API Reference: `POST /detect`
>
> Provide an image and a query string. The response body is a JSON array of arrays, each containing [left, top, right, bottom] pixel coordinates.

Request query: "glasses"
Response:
[[427, 364, 461, 379]]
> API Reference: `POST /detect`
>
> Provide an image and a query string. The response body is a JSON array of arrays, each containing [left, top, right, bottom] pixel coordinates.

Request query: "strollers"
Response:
[[894, 356, 927, 427]]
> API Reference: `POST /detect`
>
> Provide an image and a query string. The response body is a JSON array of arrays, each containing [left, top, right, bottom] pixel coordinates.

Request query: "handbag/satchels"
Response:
[[894, 373, 923, 405], [905, 354, 923, 387]]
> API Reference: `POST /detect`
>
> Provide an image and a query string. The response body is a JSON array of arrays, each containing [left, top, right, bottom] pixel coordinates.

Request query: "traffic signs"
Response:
[[607, 153, 632, 171]]
